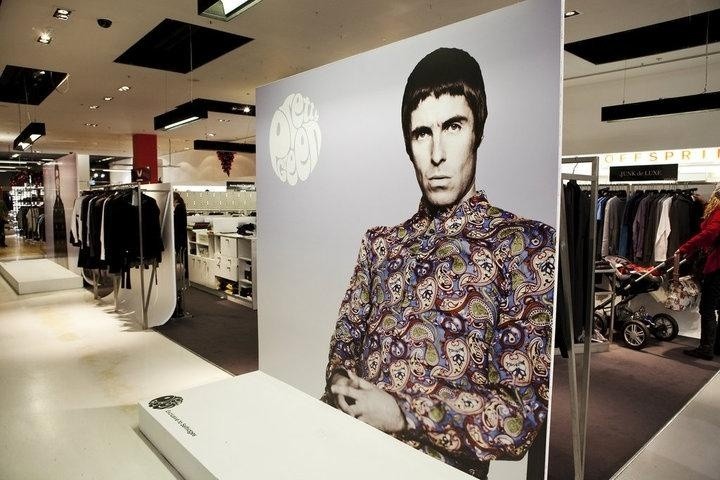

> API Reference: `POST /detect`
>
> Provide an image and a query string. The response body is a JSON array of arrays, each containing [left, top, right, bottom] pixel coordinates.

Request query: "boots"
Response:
[[683, 321, 717, 360]]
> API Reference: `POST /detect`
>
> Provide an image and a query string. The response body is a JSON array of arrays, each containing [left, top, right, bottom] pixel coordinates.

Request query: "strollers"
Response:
[[593, 248, 689, 347]]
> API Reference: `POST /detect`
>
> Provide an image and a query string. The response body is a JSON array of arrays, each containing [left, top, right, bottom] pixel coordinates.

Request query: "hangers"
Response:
[[78, 183, 139, 194], [579, 182, 688, 194]]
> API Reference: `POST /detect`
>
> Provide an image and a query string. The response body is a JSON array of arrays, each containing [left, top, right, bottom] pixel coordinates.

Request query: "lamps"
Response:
[[154, 70, 256, 155], [13, 93, 46, 152], [601, 29, 716, 124], [197, 0, 262, 22]]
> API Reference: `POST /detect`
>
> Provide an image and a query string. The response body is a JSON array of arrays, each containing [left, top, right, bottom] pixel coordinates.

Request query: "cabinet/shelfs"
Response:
[[188, 225, 253, 304]]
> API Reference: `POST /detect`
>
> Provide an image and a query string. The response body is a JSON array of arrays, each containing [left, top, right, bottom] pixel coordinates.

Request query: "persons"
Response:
[[672, 182, 720, 360], [318, 47, 555, 480], [173, 192, 187, 264]]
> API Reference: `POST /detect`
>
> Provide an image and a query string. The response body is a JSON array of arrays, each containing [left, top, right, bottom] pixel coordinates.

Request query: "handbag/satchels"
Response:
[[664, 275, 702, 312]]
